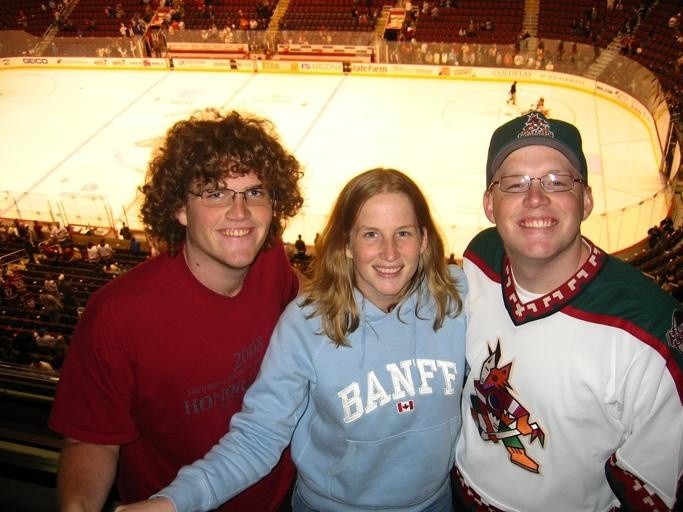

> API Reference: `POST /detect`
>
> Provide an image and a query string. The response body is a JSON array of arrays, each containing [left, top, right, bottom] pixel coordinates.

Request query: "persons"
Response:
[[296, 235, 307, 264], [537, 97, 546, 109], [646, 214, 682, 300], [0, 219, 136, 375], [16, 1, 647, 70], [449, 110, 682, 512], [46, 111, 313, 512], [114, 167, 468, 512], [448, 252, 457, 263], [508, 81, 518, 105]]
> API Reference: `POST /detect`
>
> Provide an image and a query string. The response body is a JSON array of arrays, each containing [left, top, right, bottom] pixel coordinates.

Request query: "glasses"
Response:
[[488, 173, 587, 193], [187, 187, 276, 208]]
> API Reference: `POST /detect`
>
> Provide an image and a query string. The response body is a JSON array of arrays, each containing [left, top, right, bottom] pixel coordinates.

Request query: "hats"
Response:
[[486, 111, 588, 190]]
